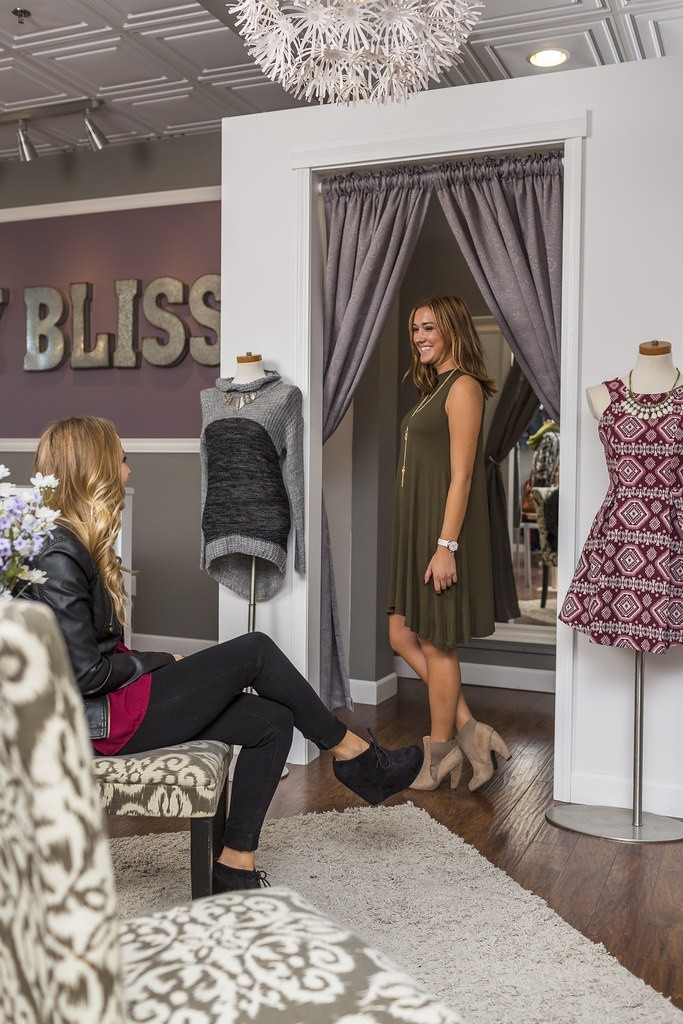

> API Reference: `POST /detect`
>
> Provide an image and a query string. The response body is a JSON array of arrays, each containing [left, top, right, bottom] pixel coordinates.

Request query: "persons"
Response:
[[11, 415, 424, 894], [545, 489, 559, 551], [200, 352, 306, 601], [558, 339, 683, 654], [387, 295, 522, 790]]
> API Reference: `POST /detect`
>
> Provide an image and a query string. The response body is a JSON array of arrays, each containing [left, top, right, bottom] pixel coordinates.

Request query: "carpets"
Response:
[[105, 801, 683, 1024]]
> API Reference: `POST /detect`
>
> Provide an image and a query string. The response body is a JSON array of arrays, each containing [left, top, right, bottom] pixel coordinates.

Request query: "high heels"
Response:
[[332, 727, 422, 805], [454, 716, 513, 791], [211, 859, 271, 896], [407, 736, 462, 791]]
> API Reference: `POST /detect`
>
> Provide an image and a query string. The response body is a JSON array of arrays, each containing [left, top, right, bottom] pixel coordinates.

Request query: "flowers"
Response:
[[0, 465, 63, 601]]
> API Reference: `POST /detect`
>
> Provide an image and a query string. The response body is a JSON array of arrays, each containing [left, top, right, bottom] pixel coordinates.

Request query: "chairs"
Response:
[[0, 600, 469, 1024], [92, 740, 234, 903]]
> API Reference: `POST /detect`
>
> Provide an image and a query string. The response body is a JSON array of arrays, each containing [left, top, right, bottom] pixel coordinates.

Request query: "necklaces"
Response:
[[402, 366, 460, 487], [621, 368, 680, 420]]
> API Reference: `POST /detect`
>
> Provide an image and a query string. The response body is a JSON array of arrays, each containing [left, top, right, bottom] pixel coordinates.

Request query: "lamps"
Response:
[[83, 109, 109, 152], [17, 120, 39, 162]]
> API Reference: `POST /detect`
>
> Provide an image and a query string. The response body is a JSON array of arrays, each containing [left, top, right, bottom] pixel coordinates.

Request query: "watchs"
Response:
[[438, 538, 459, 553]]
